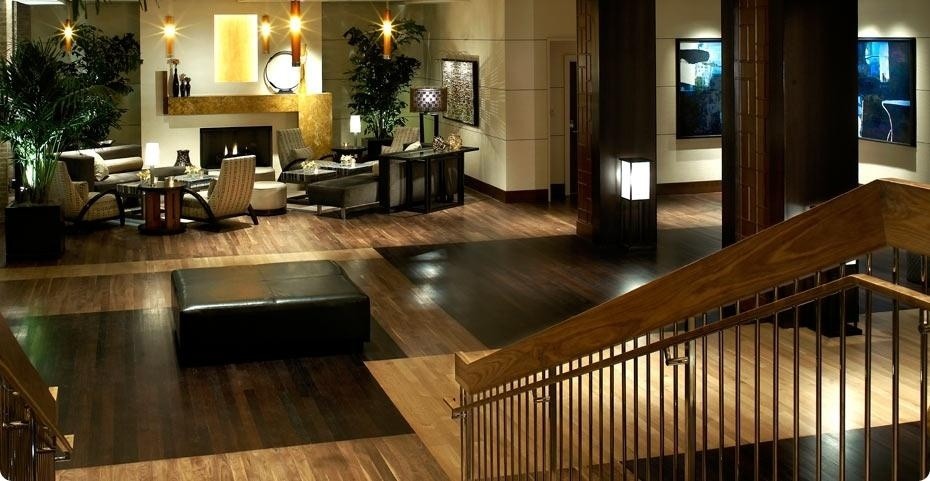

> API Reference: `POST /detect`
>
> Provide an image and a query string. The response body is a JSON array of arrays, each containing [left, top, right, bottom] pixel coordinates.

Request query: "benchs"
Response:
[[171, 258, 371, 361]]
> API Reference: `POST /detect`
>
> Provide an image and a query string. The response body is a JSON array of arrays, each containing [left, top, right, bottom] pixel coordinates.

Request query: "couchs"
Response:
[[276, 127, 338, 171], [47, 160, 125, 232], [180, 154, 259, 234], [48, 143, 144, 193], [305, 142, 432, 218]]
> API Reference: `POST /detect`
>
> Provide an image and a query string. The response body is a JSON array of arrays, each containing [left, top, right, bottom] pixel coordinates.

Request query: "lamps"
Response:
[[64, 18, 73, 52], [619, 156, 652, 202], [349, 115, 362, 148], [290, 1, 301, 68], [163, 15, 174, 59], [408, 86, 448, 143], [261, 14, 270, 54], [380, 1, 392, 61]]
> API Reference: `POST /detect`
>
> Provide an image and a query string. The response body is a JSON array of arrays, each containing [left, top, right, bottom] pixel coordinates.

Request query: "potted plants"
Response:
[[342, 17, 427, 156], [0, 37, 100, 268]]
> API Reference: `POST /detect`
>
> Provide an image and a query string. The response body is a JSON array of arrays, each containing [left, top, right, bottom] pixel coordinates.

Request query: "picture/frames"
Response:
[[440, 58, 479, 127]]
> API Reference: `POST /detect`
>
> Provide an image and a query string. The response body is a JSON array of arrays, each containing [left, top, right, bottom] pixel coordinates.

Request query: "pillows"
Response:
[[391, 127, 419, 148], [404, 140, 422, 151], [79, 149, 109, 182], [380, 145, 396, 154]]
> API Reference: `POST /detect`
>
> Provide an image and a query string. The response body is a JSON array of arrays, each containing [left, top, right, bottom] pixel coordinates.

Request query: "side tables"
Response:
[[137, 181, 186, 236], [281, 169, 336, 206], [330, 145, 367, 162]]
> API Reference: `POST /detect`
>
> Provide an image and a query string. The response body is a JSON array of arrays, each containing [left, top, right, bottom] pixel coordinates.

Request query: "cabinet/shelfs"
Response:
[[155, 70, 333, 159]]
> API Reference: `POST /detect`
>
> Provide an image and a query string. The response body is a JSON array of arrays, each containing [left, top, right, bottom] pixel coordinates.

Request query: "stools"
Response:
[[250, 180, 287, 217], [254, 165, 275, 183]]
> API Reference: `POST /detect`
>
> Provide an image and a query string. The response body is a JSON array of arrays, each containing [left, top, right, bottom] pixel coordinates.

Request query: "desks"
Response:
[[390, 143, 479, 213]]
